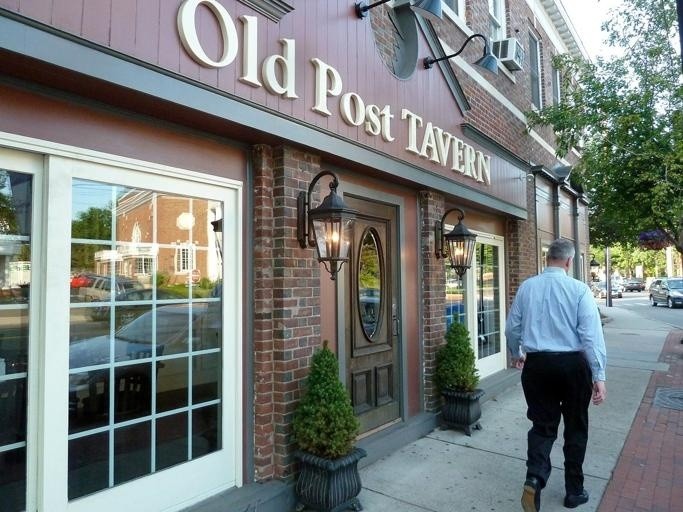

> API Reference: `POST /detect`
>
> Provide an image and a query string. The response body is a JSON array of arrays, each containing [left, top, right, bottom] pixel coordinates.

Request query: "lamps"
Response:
[[434, 208, 477, 280], [355, 0, 443, 23], [423, 34, 499, 76], [297, 171, 358, 280]]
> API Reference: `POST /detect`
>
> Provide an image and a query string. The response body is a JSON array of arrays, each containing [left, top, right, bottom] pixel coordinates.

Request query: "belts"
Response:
[[526, 351, 585, 357]]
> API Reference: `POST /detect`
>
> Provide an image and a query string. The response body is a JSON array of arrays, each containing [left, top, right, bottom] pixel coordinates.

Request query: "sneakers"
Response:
[[518, 477, 542, 512], [563, 489, 589, 508]]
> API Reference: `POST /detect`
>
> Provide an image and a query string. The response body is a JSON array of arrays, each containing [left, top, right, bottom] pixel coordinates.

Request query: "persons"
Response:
[[504, 238, 608, 512]]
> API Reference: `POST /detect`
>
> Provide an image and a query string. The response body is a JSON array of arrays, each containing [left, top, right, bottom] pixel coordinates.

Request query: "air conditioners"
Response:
[[490, 37, 526, 72]]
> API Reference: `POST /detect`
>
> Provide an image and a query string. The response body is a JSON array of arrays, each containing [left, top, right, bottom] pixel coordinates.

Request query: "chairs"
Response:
[[12, 342, 166, 443]]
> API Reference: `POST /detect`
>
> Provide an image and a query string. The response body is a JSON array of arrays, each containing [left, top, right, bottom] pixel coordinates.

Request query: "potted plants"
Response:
[[433, 313, 483, 436], [288, 340, 367, 512]]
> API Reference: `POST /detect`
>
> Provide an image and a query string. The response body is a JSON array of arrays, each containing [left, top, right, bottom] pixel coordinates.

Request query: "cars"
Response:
[[591, 273, 683, 308], [359, 287, 381, 305]]
